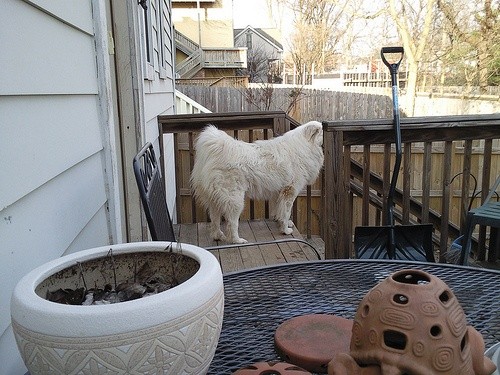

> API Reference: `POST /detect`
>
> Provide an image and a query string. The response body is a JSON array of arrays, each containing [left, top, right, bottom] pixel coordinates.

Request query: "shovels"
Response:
[[353, 45, 437, 264]]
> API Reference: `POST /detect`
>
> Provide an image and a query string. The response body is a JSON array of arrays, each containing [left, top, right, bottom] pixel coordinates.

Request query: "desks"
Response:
[[208, 258, 500, 375]]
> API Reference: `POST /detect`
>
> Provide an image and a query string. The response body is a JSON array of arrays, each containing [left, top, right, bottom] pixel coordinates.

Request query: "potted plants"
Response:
[[9, 235, 225, 375]]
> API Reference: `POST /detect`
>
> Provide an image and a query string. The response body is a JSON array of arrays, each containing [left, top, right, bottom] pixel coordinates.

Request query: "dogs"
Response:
[[187, 120, 324, 244]]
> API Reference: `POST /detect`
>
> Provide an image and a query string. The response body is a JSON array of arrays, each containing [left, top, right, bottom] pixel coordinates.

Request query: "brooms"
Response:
[[442, 173, 500, 269]]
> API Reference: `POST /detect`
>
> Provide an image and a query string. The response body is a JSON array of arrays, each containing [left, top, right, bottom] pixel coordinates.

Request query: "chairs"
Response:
[[453, 172, 500, 269], [132, 141, 324, 304]]
